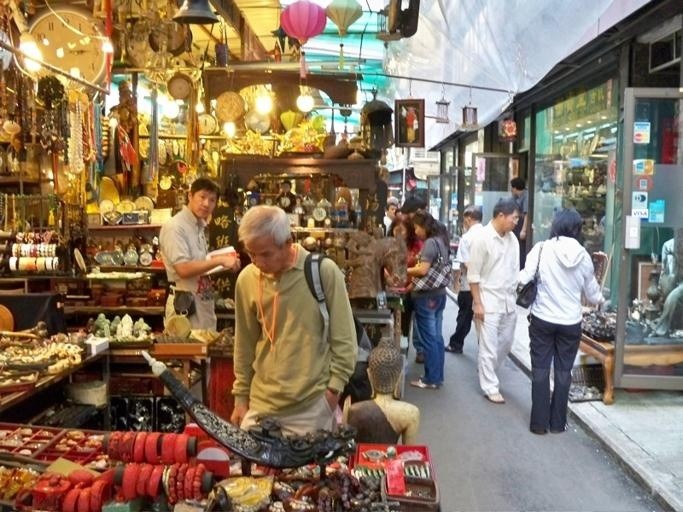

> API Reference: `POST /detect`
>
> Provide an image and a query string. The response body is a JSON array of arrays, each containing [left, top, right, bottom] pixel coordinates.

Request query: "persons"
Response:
[[276, 181, 296, 212], [342, 336, 420, 445], [228, 204, 358, 440], [159, 178, 241, 389]]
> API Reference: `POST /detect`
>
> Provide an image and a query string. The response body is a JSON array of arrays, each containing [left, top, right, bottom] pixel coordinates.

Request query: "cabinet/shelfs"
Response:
[[64, 222, 167, 318]]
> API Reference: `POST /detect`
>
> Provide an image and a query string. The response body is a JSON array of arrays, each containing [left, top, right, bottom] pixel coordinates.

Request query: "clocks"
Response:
[[21, 3, 114, 102], [119, 11, 270, 138], [239, 174, 354, 230], [80, 173, 171, 226]]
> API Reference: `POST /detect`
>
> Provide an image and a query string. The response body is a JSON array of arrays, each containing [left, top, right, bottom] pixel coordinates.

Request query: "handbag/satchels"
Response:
[[516, 279, 537, 308], [173, 291, 196, 314], [338, 314, 372, 408], [411, 237, 452, 292]]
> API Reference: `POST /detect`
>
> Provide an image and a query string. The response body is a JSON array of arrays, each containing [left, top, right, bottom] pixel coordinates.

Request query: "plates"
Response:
[[97, 196, 154, 217], [72, 248, 86, 274], [93, 249, 153, 267]]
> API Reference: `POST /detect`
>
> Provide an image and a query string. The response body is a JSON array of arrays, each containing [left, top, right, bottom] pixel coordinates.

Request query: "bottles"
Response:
[[294, 195, 363, 229]]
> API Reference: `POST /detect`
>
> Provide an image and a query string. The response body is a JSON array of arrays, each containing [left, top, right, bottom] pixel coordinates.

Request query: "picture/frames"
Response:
[[393, 99, 425, 149]]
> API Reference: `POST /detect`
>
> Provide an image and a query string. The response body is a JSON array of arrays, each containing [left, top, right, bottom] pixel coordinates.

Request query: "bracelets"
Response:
[[60, 429, 206, 511]]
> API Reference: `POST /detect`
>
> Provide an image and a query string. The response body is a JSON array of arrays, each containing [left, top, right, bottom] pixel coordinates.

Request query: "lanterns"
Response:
[[327, 0, 363, 68], [280, 0, 327, 78]]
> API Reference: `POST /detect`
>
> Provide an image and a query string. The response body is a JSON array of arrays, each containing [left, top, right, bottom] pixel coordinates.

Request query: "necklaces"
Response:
[[258, 270, 293, 353], [0, 48, 109, 271]]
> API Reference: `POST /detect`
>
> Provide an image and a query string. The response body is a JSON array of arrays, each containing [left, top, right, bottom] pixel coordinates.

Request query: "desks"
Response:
[[571, 321, 683, 405], [212, 306, 395, 346], [0, 325, 433, 510]]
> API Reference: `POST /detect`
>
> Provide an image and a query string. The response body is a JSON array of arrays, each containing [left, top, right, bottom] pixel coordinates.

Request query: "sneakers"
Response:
[[445, 345, 463, 353], [533, 427, 562, 433], [486, 394, 505, 403], [416, 353, 425, 363], [411, 376, 437, 388]]
